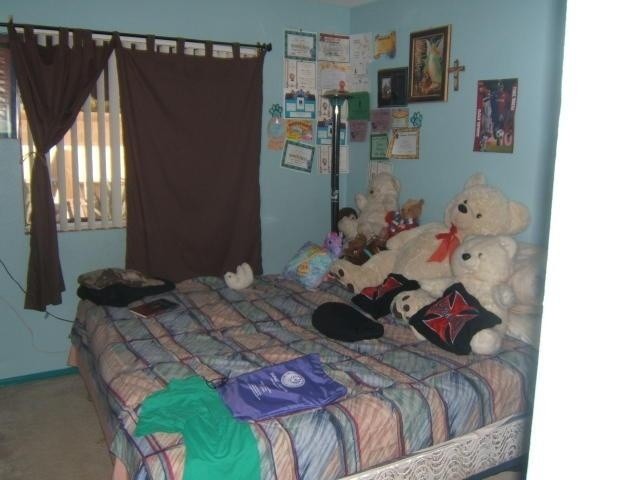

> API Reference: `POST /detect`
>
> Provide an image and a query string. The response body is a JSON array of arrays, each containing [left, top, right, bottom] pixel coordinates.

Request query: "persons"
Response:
[[475, 78, 517, 151], [451, 65, 462, 91]]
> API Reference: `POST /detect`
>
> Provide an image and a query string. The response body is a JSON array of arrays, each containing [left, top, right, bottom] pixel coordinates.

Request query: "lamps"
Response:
[[321, 87, 354, 233]]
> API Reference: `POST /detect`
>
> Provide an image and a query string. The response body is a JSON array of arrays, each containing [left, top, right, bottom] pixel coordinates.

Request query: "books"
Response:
[[130, 298, 179, 319]]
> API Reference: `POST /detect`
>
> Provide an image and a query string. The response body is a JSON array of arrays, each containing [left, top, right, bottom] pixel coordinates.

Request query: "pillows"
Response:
[[351, 272, 503, 357], [279, 240, 337, 291]]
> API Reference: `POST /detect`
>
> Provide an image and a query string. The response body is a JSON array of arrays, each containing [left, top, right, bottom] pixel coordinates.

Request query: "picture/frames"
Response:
[[377, 24, 452, 109]]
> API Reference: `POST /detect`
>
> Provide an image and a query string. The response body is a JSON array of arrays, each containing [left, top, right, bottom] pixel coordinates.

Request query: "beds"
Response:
[[77, 251, 538, 480]]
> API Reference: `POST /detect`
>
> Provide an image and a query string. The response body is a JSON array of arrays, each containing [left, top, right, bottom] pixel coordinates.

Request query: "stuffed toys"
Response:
[[226, 263, 254, 289], [329, 171, 532, 355]]
